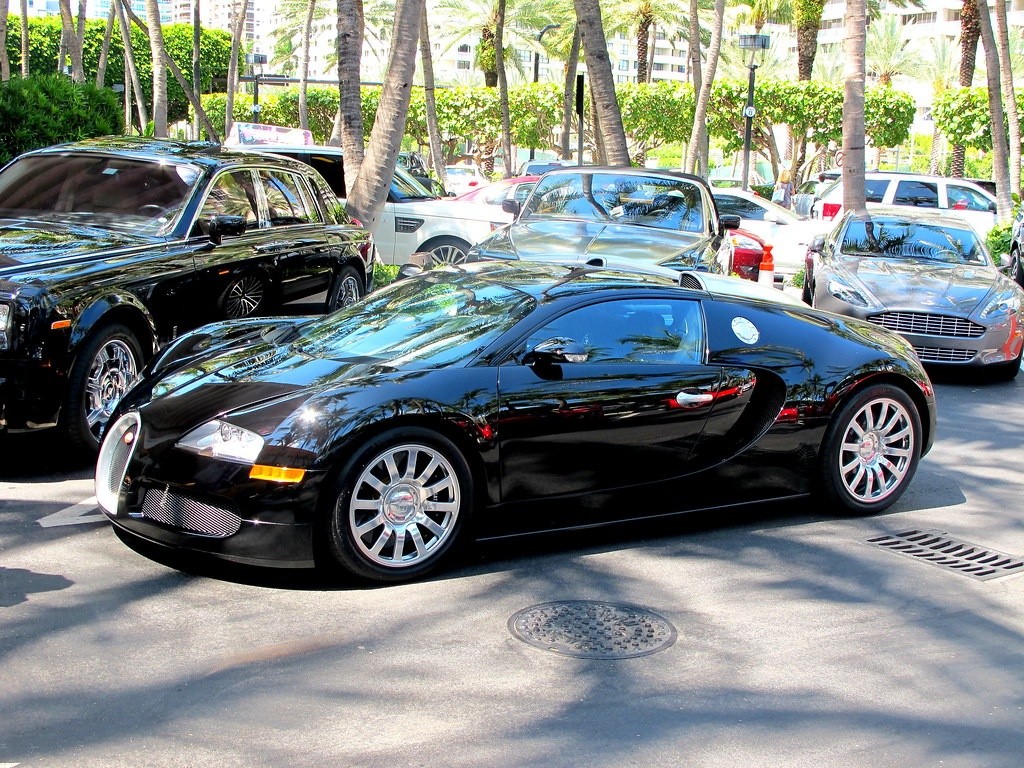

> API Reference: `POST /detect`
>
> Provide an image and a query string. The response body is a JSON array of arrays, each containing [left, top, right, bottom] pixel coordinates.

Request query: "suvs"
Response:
[[1, 136, 377, 463]]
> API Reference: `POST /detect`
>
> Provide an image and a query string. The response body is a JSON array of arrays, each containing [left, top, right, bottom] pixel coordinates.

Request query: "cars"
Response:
[[91, 262, 938, 590], [230, 144, 1024, 293], [797, 210, 1022, 386]]
